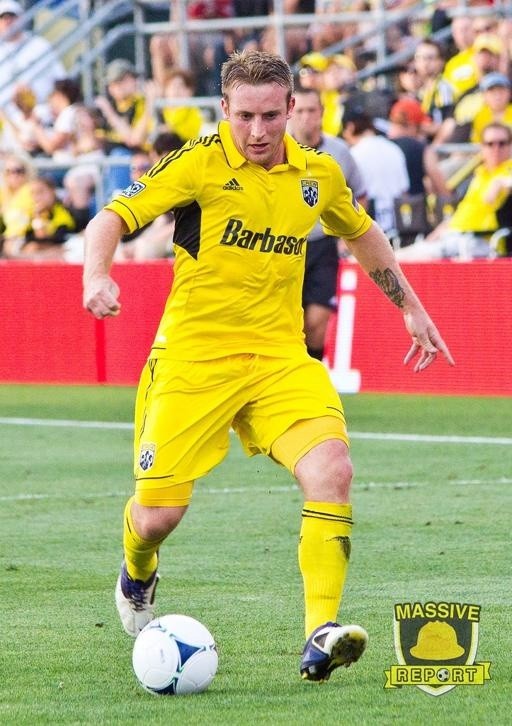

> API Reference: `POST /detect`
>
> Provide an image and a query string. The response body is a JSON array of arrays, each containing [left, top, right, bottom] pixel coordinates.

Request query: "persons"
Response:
[[82, 50, 458, 683], [0, 0, 512, 262], [286, 86, 368, 361]]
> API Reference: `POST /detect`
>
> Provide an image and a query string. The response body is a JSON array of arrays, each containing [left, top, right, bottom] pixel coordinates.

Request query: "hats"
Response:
[[0, 0, 512, 126]]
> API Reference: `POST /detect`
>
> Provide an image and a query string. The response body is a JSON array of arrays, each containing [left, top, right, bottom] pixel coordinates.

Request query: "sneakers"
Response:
[[115, 547, 161, 638], [299, 621, 369, 683]]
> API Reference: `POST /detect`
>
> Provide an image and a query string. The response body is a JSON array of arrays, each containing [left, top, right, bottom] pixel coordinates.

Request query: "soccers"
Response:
[[132, 614, 218, 695]]
